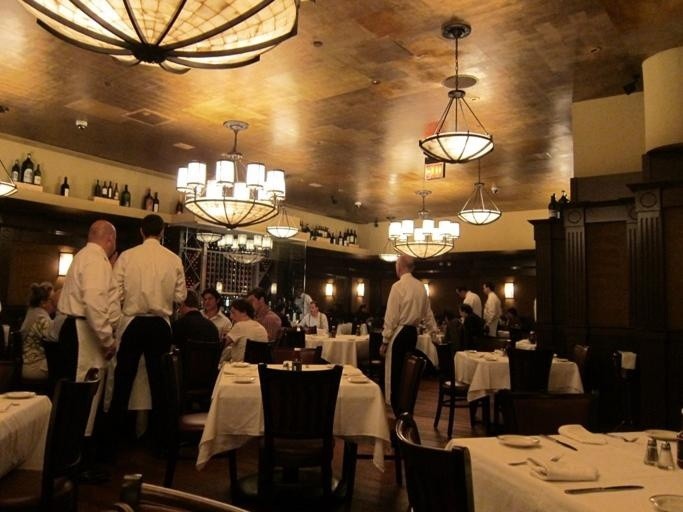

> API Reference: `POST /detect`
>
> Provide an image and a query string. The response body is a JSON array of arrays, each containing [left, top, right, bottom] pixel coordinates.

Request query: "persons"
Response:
[[44, 218, 121, 486], [16, 282, 62, 379], [170, 287, 386, 447], [105, 214, 188, 462], [432, 280, 525, 379], [378, 255, 440, 418]]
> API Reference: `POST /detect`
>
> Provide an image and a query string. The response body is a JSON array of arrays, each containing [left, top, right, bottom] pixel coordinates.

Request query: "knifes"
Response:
[[564, 485, 643, 494]]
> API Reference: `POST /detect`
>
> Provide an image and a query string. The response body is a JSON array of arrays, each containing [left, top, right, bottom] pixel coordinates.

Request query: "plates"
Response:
[[644, 429, 677, 440], [229, 377, 254, 383], [348, 376, 369, 384], [7, 391, 35, 398], [650, 494, 682, 511], [496, 433, 539, 447]]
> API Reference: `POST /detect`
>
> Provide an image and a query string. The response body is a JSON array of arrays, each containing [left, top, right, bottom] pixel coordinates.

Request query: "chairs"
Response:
[[393, 412, 474, 511], [392, 309, 650, 430], [2, 281, 393, 512]]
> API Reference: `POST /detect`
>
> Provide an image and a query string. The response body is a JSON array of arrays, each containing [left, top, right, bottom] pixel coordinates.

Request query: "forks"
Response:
[[541, 432, 577, 452], [597, 428, 639, 447]]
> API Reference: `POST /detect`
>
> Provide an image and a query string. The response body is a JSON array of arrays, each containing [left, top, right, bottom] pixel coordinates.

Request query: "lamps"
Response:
[[18, 0, 299, 74], [266, 204, 299, 238], [217, 230, 273, 264], [196, 233, 221, 248], [0, 160, 19, 206], [458, 158, 499, 226], [379, 216, 401, 263], [173, 122, 286, 230], [387, 191, 460, 260], [419, 20, 495, 164]]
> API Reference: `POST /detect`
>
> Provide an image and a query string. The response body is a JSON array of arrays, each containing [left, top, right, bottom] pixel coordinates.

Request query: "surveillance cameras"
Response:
[[75, 120, 88, 131]]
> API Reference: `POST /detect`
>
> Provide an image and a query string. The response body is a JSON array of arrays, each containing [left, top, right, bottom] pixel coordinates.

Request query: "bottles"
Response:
[[222, 295, 239, 303], [282, 362, 289, 369], [277, 298, 299, 319], [10, 152, 41, 186], [60, 175, 69, 197], [642, 436, 658, 464], [658, 440, 673, 470], [299, 222, 358, 248], [356, 325, 359, 336], [96, 179, 118, 199], [292, 348, 302, 370], [152, 192, 159, 212], [331, 325, 336, 338], [175, 192, 182, 214], [143, 187, 153, 211], [677, 409, 682, 466], [529, 331, 536, 344], [120, 183, 131, 206]]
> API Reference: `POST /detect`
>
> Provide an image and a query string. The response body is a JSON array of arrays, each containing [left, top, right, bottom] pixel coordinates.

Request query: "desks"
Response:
[[444, 429, 682, 512]]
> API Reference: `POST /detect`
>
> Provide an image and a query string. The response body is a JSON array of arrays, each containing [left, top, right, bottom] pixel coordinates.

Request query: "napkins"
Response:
[[559, 425, 607, 445], [525, 456, 600, 482]]
